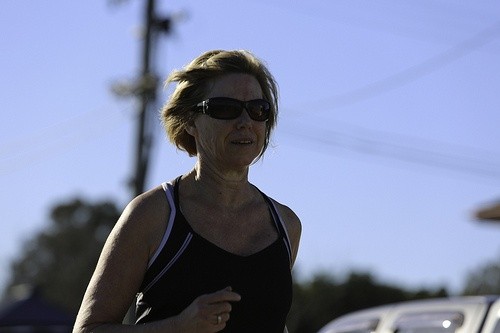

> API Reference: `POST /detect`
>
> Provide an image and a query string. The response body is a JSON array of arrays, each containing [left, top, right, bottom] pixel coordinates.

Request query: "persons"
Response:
[[71, 49, 303, 333]]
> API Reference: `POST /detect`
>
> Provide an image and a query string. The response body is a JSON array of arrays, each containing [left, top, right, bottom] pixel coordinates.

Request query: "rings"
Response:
[[217, 315, 222, 324]]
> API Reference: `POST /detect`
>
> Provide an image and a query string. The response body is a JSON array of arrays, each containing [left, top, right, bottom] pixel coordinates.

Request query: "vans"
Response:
[[318, 294, 500, 333]]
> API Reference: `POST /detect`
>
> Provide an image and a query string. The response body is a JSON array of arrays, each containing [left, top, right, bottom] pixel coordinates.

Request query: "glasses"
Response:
[[193, 97, 274, 122]]
